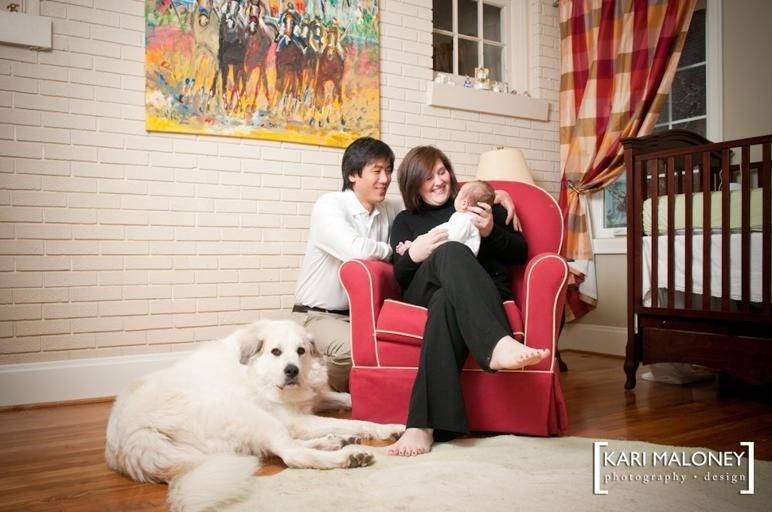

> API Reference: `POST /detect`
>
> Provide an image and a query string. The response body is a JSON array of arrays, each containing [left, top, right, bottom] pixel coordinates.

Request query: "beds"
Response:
[[620, 130, 772, 389]]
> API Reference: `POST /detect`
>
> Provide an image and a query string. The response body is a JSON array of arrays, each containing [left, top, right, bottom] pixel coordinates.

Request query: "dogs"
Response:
[[102, 318, 407, 512]]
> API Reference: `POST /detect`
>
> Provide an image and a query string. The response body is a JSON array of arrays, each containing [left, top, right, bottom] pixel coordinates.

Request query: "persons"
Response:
[[384, 146, 552, 458], [290, 136, 524, 392], [395, 181, 495, 264]]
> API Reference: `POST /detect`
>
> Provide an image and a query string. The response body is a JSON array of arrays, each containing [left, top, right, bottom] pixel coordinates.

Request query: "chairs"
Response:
[[339, 181, 568, 436]]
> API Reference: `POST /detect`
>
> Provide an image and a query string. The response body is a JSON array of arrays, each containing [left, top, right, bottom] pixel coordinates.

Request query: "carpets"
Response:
[[202, 433, 771, 511]]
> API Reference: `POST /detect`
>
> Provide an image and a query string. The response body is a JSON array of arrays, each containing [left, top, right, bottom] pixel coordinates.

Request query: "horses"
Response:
[[178, 0, 346, 127]]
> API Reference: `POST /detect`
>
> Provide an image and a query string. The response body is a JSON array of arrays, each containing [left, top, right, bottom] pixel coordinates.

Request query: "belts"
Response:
[[291, 305, 350, 315]]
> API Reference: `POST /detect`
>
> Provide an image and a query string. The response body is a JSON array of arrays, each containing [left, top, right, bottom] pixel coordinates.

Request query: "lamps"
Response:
[[476, 147, 534, 186]]
